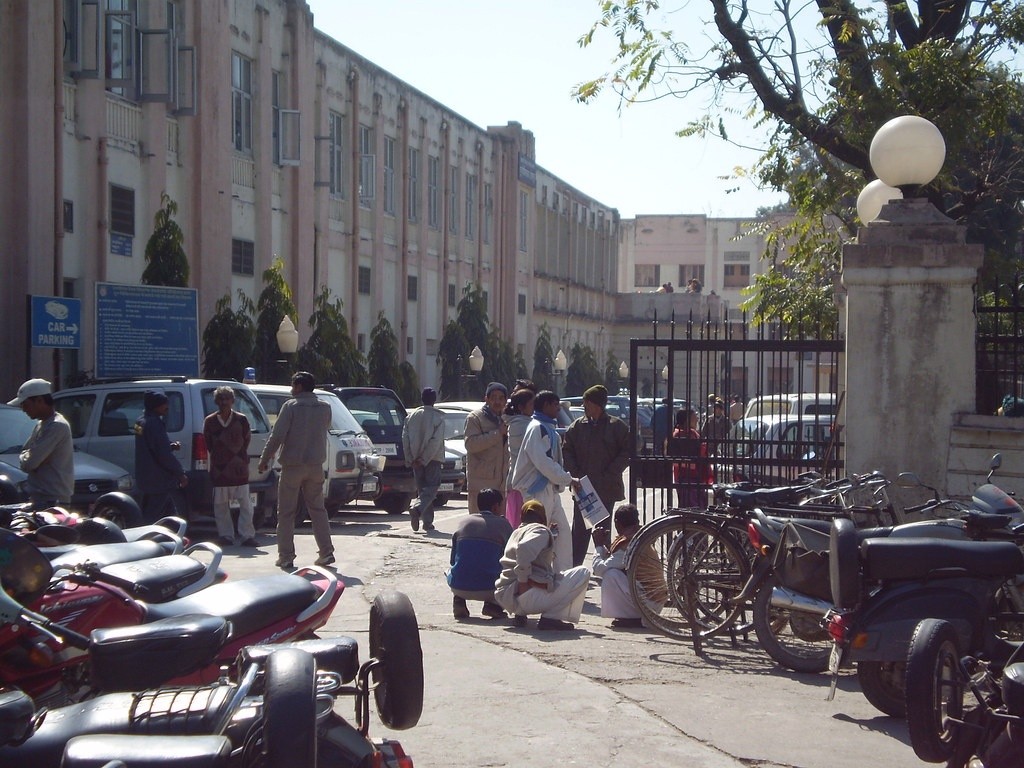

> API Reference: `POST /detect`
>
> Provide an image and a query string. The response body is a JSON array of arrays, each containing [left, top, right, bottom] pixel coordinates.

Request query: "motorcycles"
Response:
[[728, 452, 1024, 768], [1, 475, 424, 767]]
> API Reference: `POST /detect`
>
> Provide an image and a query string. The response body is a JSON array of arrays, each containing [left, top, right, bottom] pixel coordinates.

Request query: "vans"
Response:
[[240, 384, 386, 529], [745, 392, 838, 417]]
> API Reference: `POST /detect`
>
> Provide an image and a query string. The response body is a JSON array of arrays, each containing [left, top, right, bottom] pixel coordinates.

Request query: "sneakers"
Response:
[[276, 559, 293, 568], [315, 553, 335, 565]]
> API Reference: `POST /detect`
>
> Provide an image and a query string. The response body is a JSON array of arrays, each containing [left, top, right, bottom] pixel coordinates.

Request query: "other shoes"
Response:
[[454, 607, 469, 617], [242, 538, 261, 546], [423, 524, 435, 530], [483, 602, 507, 619], [515, 614, 528, 625], [215, 537, 233, 546], [538, 619, 574, 631], [612, 618, 641, 628], [409, 507, 420, 531]]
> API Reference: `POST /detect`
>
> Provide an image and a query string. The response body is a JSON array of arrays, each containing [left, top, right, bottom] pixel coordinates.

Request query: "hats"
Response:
[[486, 381, 507, 398], [7, 378, 50, 406], [422, 388, 436, 402], [584, 385, 608, 409], [149, 393, 167, 408]]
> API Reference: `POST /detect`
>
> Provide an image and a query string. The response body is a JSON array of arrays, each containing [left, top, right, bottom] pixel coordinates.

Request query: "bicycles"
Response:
[[621, 470, 970, 657]]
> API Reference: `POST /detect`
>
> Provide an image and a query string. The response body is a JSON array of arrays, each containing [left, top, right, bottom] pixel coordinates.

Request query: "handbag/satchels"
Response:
[[772, 519, 833, 603]]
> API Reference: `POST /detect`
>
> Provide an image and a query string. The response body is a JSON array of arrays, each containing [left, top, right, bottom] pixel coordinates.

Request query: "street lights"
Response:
[[456, 345, 485, 403], [610, 361, 629, 396], [661, 365, 669, 399], [545, 348, 567, 400], [262, 315, 299, 386]]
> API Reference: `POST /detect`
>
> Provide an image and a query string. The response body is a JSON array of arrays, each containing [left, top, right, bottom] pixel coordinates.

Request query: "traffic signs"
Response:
[[29, 294, 83, 350]]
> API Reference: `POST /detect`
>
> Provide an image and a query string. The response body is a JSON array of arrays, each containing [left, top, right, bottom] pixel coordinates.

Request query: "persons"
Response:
[[699, 394, 751, 456], [656, 282, 673, 293], [664, 409, 714, 552], [512, 391, 580, 573], [463, 379, 538, 515], [494, 500, 591, 629], [562, 385, 641, 567], [402, 388, 446, 531], [7, 379, 74, 511], [135, 391, 187, 533], [258, 373, 335, 568], [686, 279, 703, 294], [204, 386, 260, 547], [711, 290, 715, 295], [651, 399, 676, 455], [592, 505, 667, 627], [447, 487, 514, 618]]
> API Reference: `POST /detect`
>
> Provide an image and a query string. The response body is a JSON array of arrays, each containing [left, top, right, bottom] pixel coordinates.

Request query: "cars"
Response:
[[311, 380, 512, 516], [561, 391, 695, 450], [0, 403, 134, 517], [704, 414, 835, 506]]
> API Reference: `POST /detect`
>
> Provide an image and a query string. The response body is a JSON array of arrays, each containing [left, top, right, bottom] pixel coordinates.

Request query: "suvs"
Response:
[[49, 376, 280, 534]]
